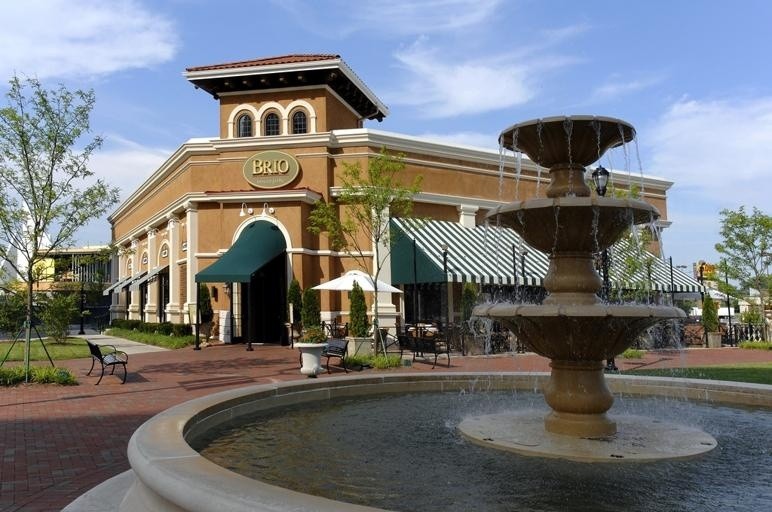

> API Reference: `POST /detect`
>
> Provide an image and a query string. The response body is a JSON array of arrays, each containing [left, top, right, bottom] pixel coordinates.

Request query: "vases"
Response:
[[290, 343, 329, 374]]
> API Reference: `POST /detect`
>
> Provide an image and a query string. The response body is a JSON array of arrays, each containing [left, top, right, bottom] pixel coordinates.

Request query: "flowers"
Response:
[[293, 330, 329, 344]]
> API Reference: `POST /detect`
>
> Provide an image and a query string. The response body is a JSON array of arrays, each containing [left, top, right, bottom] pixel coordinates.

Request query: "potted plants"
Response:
[[701, 293, 723, 347]]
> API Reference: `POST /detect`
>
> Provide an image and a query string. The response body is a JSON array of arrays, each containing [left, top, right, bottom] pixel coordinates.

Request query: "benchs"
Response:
[[85, 337, 129, 386], [394, 334, 452, 368], [298, 335, 351, 376]]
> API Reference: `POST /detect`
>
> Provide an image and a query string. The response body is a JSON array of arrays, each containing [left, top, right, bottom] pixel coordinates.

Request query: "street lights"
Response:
[[78, 256, 87, 335], [520, 249, 527, 305], [440, 244, 449, 355], [697, 259, 708, 347], [591, 164, 616, 372]]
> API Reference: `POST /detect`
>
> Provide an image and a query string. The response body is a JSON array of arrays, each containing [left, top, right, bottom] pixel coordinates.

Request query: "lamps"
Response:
[[115, 244, 130, 256], [145, 226, 165, 239], [130, 238, 145, 251], [222, 283, 229, 293], [166, 217, 186, 231], [260, 201, 275, 219], [236, 202, 255, 219]]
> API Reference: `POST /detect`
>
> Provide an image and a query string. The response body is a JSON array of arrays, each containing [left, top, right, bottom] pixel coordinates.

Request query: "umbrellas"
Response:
[[310, 268, 404, 293]]
